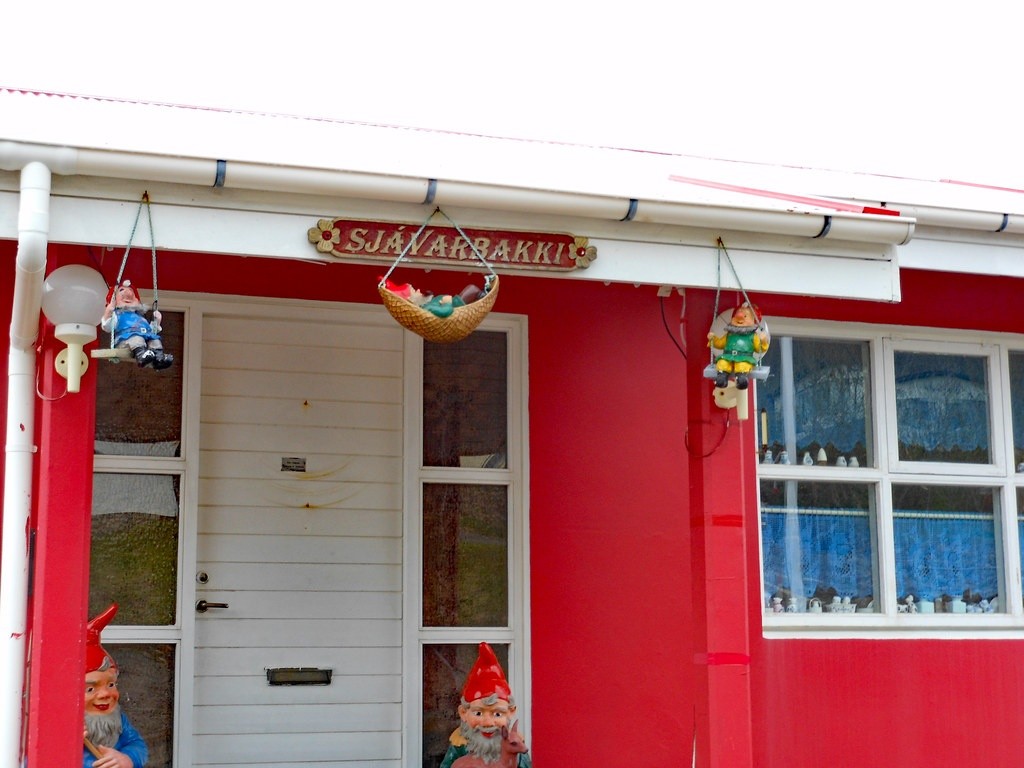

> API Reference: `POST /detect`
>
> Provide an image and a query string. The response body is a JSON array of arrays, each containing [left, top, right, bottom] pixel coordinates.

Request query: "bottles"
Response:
[[764, 449, 859, 467], [764, 595, 993, 614]]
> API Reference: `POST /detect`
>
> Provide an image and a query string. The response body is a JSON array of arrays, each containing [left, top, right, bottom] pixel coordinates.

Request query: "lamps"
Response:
[[40, 264, 110, 395], [703, 308, 771, 421]]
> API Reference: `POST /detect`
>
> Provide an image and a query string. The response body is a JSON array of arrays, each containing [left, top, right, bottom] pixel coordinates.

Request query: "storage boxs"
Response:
[[825, 603, 857, 613]]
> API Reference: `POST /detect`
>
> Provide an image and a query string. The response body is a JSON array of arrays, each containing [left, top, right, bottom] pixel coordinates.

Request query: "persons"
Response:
[[84, 604, 149, 768], [101, 284, 174, 371], [376, 274, 486, 318], [440, 641, 533, 768], [707, 302, 769, 390]]
